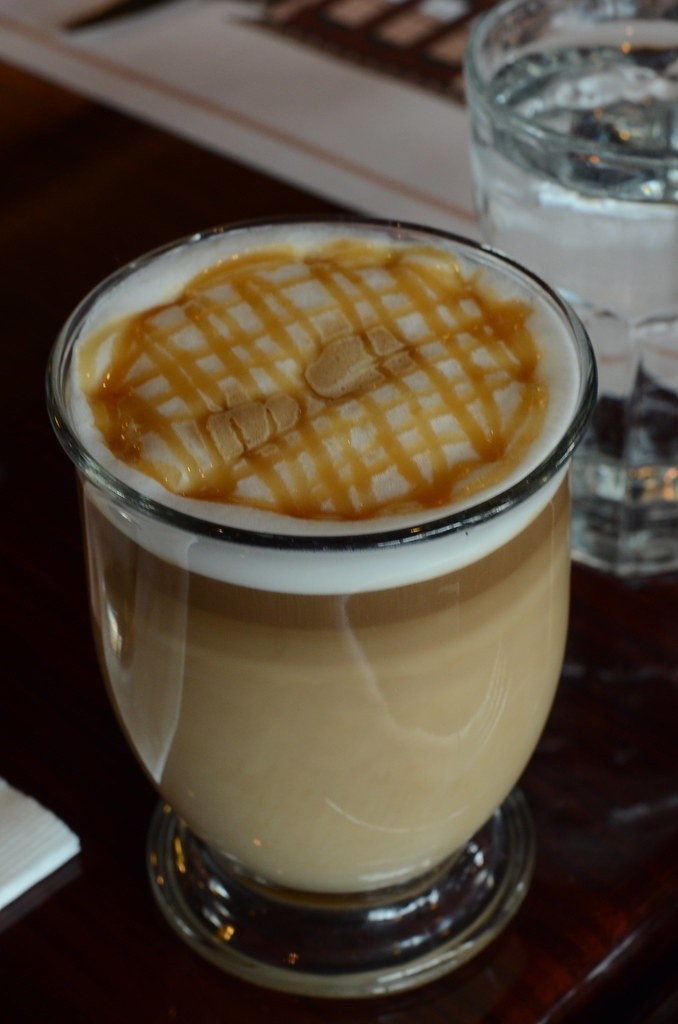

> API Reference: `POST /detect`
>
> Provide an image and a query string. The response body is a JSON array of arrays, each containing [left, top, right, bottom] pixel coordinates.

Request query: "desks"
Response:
[[0, 0, 678, 1024]]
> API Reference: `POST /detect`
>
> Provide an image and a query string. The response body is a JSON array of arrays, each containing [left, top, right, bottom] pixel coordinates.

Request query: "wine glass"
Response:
[[42, 220, 600, 1001]]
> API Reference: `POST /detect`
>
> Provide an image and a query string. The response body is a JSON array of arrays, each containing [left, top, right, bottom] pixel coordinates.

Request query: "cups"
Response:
[[462, 0, 677, 579]]
